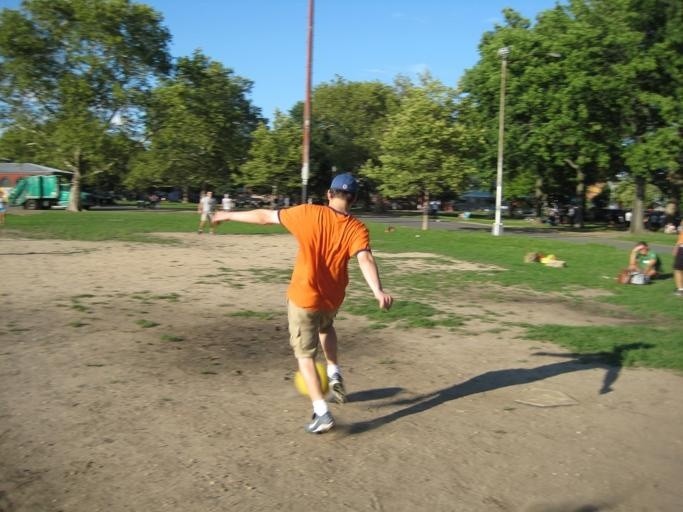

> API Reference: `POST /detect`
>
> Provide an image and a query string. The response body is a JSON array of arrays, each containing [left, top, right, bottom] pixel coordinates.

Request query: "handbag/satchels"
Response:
[[524, 252, 539, 263], [618, 270, 650, 285]]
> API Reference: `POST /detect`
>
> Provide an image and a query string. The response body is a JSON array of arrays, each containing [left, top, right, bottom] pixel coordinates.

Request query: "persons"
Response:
[[536, 254, 568, 268], [544, 202, 583, 227], [629, 241, 662, 277], [604, 199, 679, 232], [135, 191, 162, 208], [209, 173, 394, 434], [0, 187, 8, 224], [671, 219, 683, 296], [428, 199, 442, 217], [198, 189, 314, 233]]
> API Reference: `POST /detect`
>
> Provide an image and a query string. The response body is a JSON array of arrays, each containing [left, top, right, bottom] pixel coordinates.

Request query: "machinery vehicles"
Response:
[[6, 174, 95, 211]]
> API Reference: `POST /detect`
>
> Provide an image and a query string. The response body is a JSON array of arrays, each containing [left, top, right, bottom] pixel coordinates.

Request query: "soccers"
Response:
[[296, 362, 328, 396]]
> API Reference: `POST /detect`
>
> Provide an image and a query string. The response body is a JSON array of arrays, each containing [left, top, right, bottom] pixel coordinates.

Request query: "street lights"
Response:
[[491, 43, 511, 237]]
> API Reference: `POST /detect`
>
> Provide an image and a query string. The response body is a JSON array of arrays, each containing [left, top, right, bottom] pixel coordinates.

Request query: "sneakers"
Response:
[[308, 411, 336, 434], [672, 289, 683, 295], [329, 372, 347, 403]]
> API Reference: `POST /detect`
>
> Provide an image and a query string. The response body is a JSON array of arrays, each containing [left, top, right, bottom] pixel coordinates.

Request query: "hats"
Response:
[[331, 174, 357, 192]]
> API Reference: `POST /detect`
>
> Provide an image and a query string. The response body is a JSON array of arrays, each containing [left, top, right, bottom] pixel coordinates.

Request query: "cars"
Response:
[[546, 205, 678, 232], [235, 195, 264, 209]]
